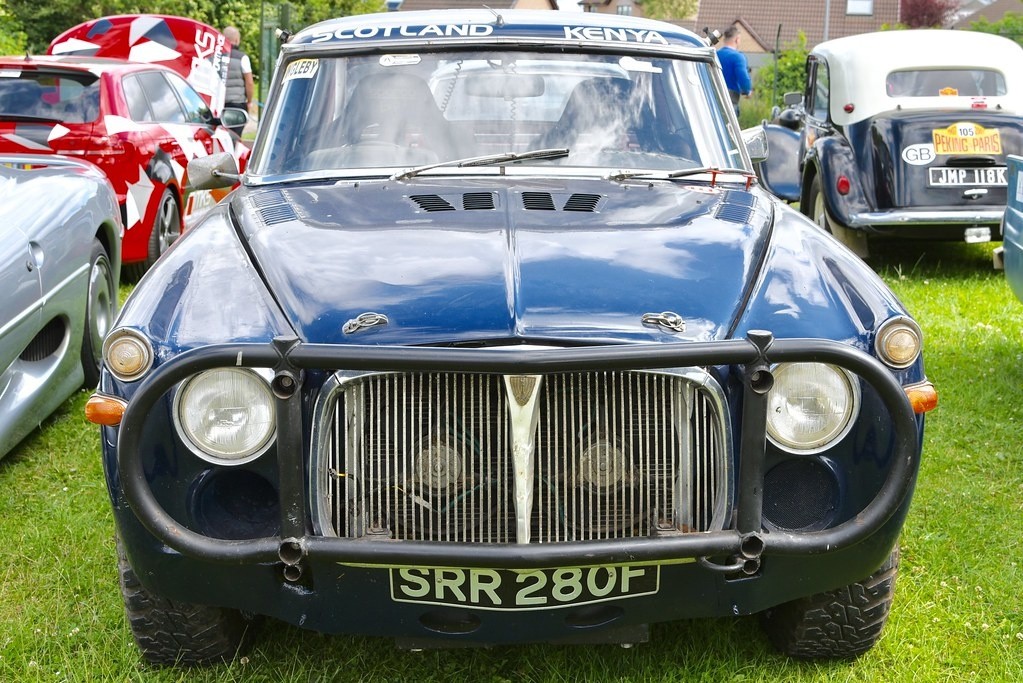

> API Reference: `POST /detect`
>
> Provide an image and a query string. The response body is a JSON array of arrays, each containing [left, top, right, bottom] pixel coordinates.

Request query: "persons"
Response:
[[223, 26, 254, 137], [716, 26, 752, 116]]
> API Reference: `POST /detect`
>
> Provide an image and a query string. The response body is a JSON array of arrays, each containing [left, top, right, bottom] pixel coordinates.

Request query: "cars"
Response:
[[1, 154, 124, 460], [83, 4, 937, 673], [0, 14, 254, 289], [781, 27, 1023, 270], [427, 57, 627, 162]]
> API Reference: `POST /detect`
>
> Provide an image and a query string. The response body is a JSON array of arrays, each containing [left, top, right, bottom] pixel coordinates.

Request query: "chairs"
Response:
[[324, 73, 462, 165], [535, 76, 693, 167]]
